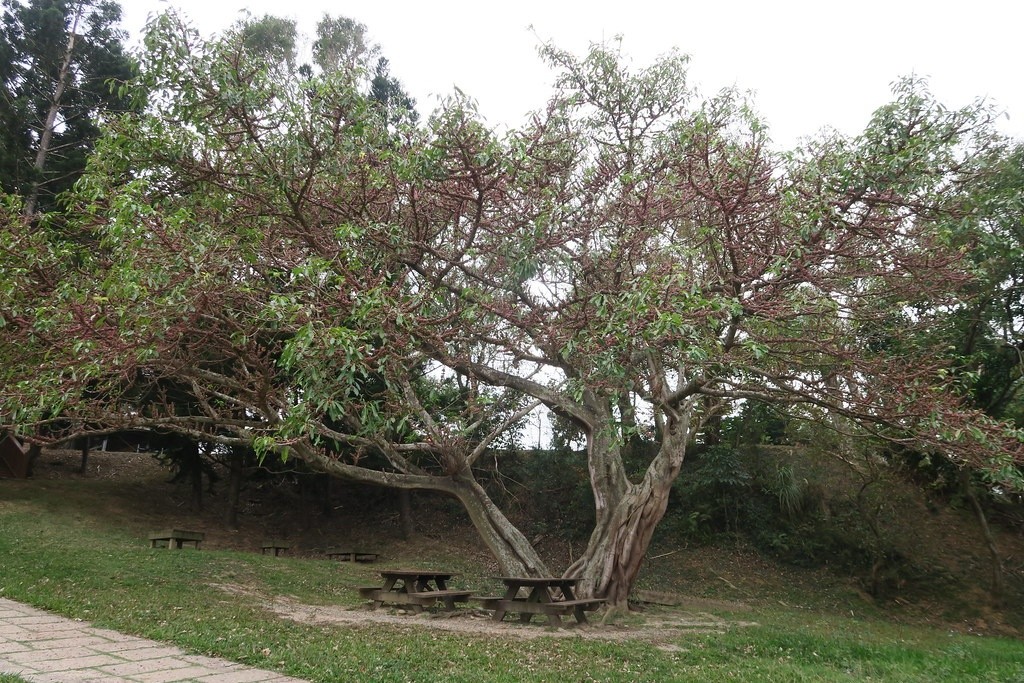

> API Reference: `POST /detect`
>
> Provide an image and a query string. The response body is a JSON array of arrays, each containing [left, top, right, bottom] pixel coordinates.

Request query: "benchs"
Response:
[[325, 548, 380, 562], [358, 587, 609, 616], [149, 529, 204, 551]]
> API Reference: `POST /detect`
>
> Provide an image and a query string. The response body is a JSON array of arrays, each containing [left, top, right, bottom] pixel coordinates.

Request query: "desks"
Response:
[[492, 577, 588, 629], [369, 570, 463, 614], [258, 540, 289, 557]]
